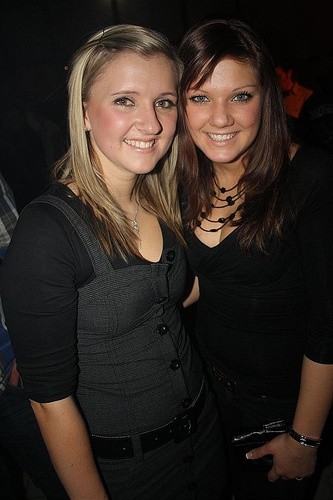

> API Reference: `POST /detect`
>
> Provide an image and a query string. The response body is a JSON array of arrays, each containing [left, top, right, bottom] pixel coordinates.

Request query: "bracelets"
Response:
[[288, 426, 321, 449]]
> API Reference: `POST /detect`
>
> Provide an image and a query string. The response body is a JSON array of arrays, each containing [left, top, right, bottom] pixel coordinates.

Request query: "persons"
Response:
[[0, 171, 57, 500], [1, 24, 217, 500], [274, 51, 333, 143], [174, 21, 333, 500]]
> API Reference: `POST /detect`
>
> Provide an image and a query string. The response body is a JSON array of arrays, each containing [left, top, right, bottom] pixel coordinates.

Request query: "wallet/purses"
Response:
[[235, 420, 327, 476]]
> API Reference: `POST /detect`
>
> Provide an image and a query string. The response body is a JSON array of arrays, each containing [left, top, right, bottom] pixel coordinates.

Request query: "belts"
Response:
[[202, 357, 278, 404], [88, 374, 207, 459]]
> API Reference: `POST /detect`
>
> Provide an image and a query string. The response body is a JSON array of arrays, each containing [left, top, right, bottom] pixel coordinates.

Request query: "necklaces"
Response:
[[196, 172, 246, 234], [126, 202, 139, 232]]
